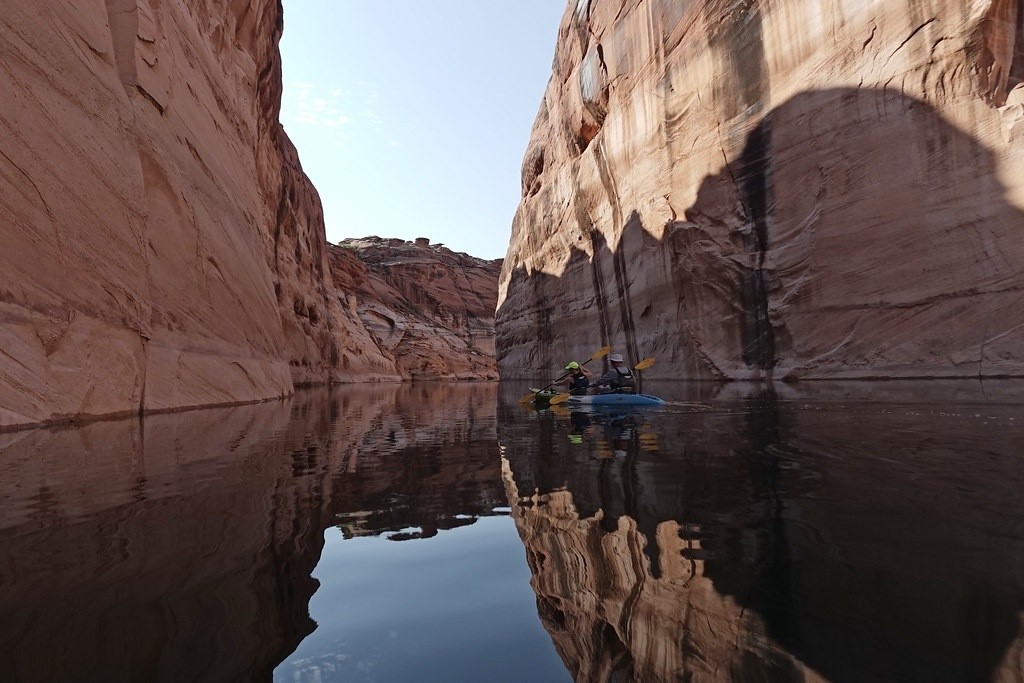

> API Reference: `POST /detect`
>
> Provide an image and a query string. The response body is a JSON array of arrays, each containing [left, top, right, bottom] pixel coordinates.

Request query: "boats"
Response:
[[528, 387, 668, 405]]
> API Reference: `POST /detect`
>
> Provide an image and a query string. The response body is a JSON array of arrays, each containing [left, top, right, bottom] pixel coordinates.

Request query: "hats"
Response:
[[607, 353, 623, 362], [565, 361, 579, 369]]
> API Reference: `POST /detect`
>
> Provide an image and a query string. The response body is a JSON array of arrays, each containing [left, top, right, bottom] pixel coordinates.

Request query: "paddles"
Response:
[[548, 358, 655, 405], [519, 346, 610, 403]]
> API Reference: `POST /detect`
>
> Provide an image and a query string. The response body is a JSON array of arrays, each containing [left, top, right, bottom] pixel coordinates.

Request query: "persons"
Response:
[[551, 362, 592, 395], [589, 354, 636, 394]]
